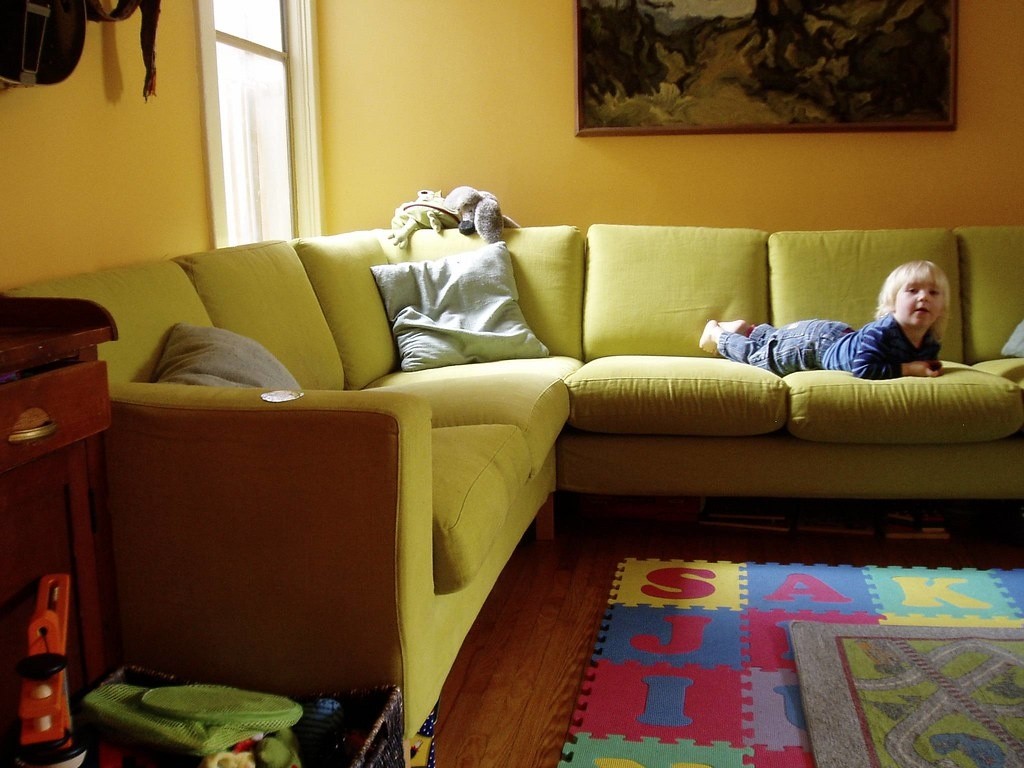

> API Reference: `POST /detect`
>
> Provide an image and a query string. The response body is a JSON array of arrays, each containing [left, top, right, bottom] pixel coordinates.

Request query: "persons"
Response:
[[699, 260, 952, 380]]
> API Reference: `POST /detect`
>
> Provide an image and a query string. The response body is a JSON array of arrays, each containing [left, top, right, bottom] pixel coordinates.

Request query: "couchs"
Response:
[[0, 222, 1024, 768]]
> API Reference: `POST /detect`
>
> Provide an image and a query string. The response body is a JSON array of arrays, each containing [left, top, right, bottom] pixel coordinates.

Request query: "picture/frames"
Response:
[[571, 0, 961, 137]]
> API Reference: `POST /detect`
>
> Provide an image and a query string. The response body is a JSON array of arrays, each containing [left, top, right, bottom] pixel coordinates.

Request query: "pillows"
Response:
[[151, 324, 301, 392], [371, 241, 549, 372]]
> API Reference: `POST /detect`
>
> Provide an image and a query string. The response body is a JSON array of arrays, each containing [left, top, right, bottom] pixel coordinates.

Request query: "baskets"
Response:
[[99, 665, 405, 768]]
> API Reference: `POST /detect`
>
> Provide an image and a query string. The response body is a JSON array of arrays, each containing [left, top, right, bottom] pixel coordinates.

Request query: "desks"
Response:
[[0, 294, 118, 731]]
